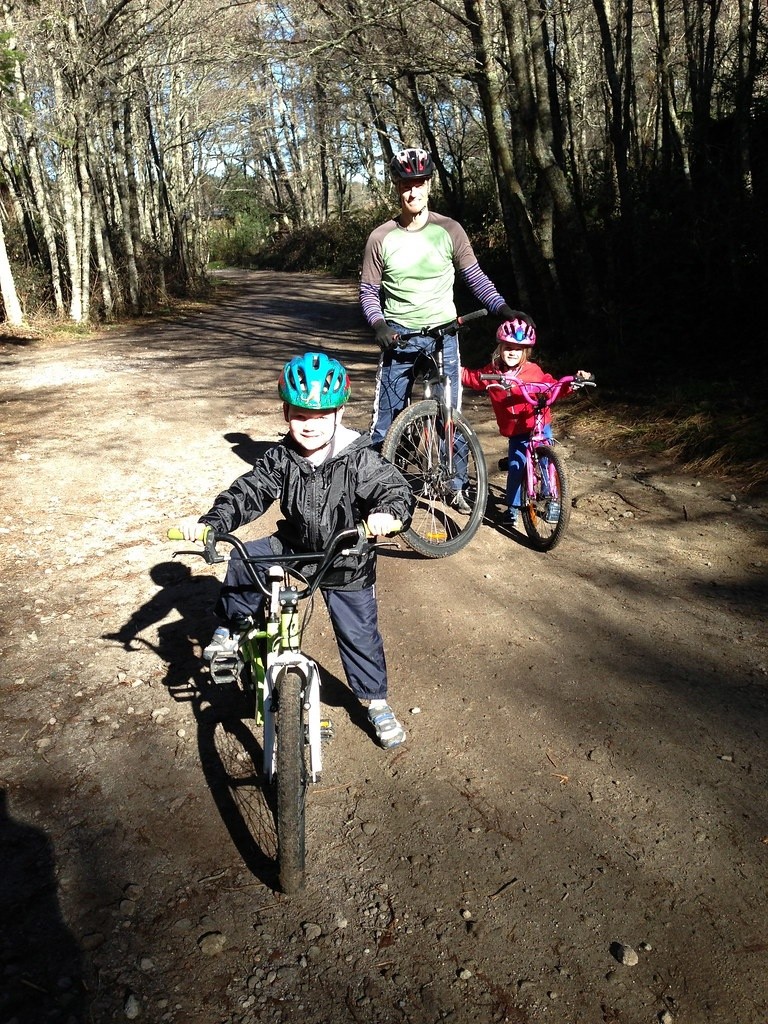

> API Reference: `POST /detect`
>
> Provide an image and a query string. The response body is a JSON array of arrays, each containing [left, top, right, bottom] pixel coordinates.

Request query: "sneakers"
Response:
[[504, 507, 519, 524], [365, 705, 406, 748], [545, 501, 561, 524], [203, 626, 257, 659], [451, 488, 476, 514]]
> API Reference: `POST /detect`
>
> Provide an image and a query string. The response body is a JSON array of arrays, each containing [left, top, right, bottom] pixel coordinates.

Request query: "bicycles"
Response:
[[381, 308, 490, 559], [166, 520, 405, 896], [479, 373, 597, 553]]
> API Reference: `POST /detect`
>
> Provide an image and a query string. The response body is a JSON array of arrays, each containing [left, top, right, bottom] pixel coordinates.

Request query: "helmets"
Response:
[[278, 353, 351, 409], [497, 319, 536, 346], [389, 148, 434, 182]]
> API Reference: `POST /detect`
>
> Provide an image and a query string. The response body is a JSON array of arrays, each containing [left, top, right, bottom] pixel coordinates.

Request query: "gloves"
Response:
[[372, 320, 397, 349], [496, 304, 536, 329]]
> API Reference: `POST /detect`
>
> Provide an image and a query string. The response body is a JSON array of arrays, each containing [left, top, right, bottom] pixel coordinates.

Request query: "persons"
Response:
[[177, 353, 418, 749], [359, 149, 537, 515], [462, 319, 590, 524]]
